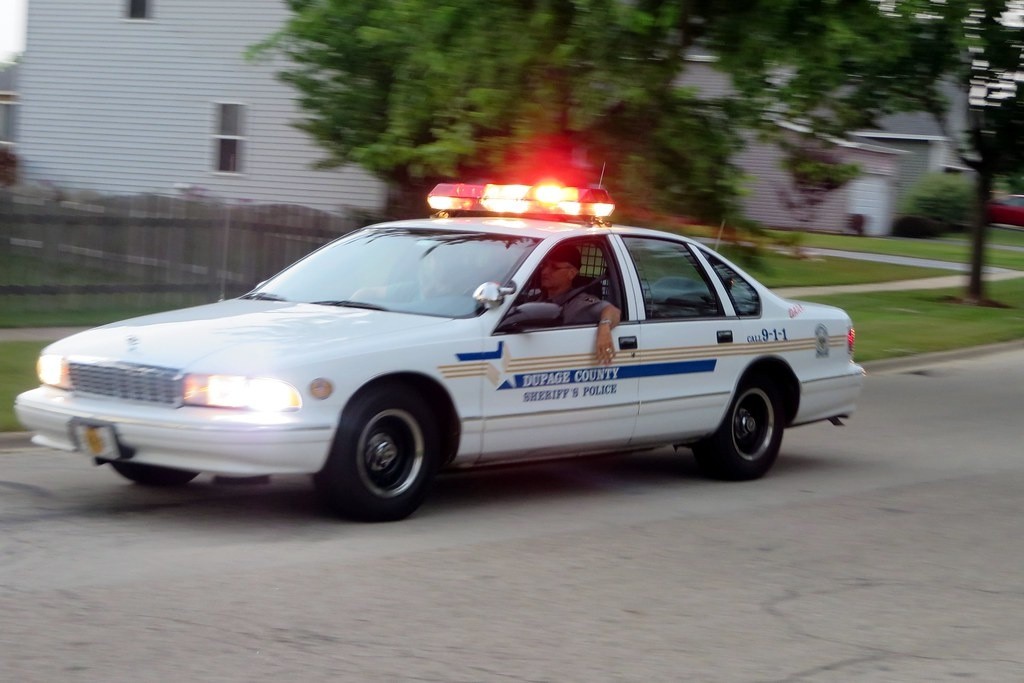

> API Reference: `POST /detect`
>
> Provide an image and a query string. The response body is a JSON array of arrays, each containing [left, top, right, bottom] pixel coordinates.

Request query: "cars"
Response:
[[986, 194, 1024, 227], [14, 183, 866, 522]]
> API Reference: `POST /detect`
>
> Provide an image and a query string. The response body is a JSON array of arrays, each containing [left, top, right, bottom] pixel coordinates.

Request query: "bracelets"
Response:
[[599, 320, 613, 327]]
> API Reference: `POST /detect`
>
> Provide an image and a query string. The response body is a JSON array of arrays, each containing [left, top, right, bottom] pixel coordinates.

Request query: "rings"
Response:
[[606, 348, 612, 353]]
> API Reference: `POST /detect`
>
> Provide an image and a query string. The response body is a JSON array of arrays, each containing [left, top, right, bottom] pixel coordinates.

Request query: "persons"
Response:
[[525, 246, 621, 367]]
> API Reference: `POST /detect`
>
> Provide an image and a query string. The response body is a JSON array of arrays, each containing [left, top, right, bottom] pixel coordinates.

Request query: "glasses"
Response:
[[545, 263, 573, 271]]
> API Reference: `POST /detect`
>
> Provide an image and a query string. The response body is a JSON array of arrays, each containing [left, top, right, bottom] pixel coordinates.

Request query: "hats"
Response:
[[545, 245, 583, 270]]
